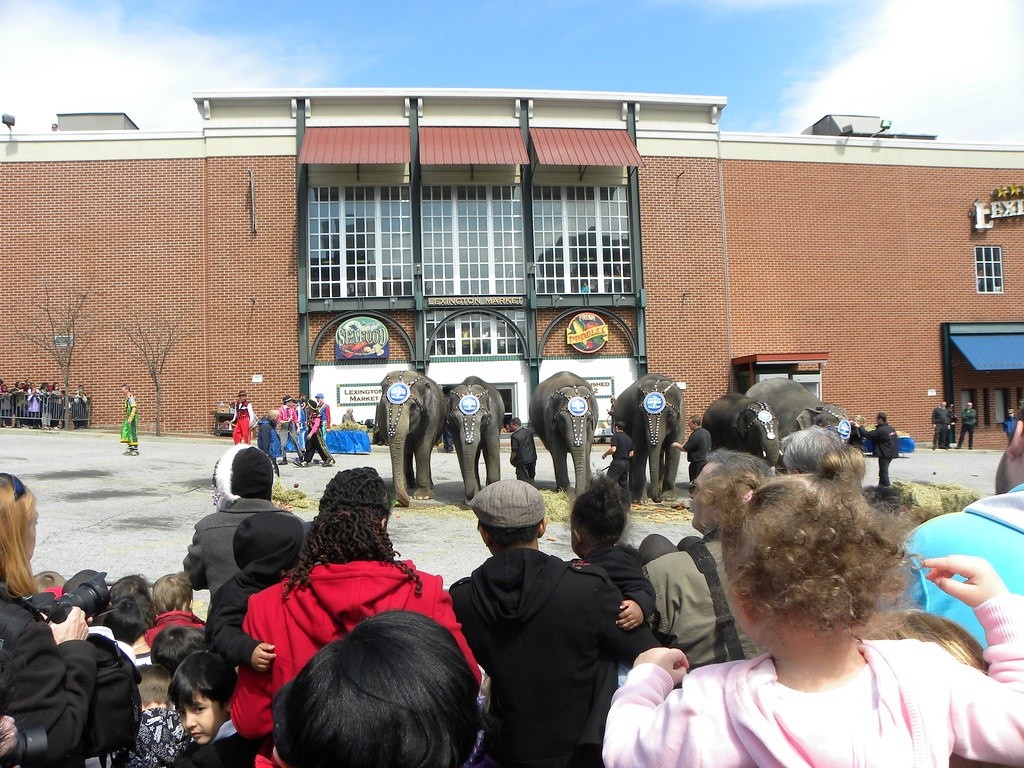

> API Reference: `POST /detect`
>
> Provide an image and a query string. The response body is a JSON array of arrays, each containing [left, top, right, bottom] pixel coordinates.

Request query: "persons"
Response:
[[932, 402, 976, 450], [231, 388, 360, 467], [121, 385, 141, 456], [1003, 408, 1017, 448], [0, 378, 88, 428], [0, 413, 1024, 768]]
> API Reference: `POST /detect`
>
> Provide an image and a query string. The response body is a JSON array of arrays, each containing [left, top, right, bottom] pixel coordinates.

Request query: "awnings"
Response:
[[950, 335, 1024, 371]]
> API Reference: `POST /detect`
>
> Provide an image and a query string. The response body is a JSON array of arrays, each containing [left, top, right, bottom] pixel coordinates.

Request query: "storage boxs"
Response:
[[218, 406, 235, 414]]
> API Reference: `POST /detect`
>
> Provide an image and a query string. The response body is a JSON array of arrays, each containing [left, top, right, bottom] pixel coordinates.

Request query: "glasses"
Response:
[[1008, 412, 1014, 415], [0, 472, 26, 500], [687, 481, 703, 497]]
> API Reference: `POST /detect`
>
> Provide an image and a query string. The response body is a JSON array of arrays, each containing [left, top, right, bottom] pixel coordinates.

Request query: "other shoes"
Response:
[[322, 460, 335, 466], [123, 448, 133, 455], [278, 460, 288, 465], [3, 418, 87, 430], [130, 450, 139, 455], [932, 443, 973, 450], [299, 457, 303, 463], [299, 460, 310, 467]]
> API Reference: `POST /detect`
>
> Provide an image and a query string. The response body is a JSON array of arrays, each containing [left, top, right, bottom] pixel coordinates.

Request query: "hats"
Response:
[[315, 393, 324, 398], [283, 395, 292, 402], [307, 399, 317, 410], [211, 444, 274, 508], [238, 391, 247, 396], [470, 479, 546, 529]]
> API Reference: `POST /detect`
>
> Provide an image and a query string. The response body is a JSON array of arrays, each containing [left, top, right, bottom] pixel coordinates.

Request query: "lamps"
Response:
[[2, 114, 14, 132], [873, 121, 892, 137], [842, 125, 853, 138]]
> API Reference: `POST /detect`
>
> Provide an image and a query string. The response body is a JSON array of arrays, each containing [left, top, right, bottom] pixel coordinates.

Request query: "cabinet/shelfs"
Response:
[[211, 411, 236, 436]]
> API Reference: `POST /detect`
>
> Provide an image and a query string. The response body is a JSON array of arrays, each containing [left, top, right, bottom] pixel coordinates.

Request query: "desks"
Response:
[[286, 430, 371, 454], [864, 438, 915, 458]]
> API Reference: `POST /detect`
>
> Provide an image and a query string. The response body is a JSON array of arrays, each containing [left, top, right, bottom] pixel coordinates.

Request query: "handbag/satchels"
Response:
[[66, 633, 143, 759]]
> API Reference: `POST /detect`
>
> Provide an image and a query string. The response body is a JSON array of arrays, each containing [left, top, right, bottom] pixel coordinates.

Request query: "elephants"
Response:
[[449, 376, 504, 504], [702, 393, 779, 468], [378, 371, 448, 508], [746, 378, 849, 446], [529, 371, 599, 498], [615, 373, 683, 503]]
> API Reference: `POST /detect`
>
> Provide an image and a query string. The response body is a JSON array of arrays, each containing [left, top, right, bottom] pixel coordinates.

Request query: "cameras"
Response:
[[0, 725, 48, 768], [24, 572, 118, 624]]
